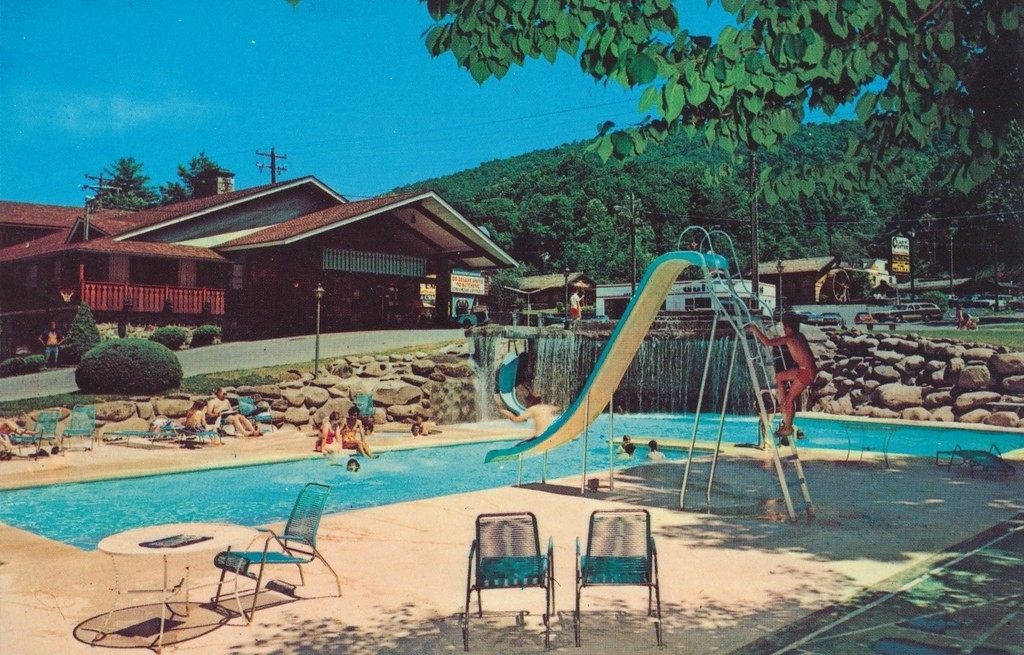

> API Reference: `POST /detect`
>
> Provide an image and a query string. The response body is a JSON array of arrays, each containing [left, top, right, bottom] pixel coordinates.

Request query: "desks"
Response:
[[90, 522, 258, 654], [842, 424, 897, 469]]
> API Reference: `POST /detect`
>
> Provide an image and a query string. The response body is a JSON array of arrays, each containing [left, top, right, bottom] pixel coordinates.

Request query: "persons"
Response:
[[39, 322, 67, 370], [955, 306, 978, 331], [0, 418, 39, 451], [570, 290, 584, 320], [500, 391, 560, 447], [753, 392, 805, 446], [751, 312, 817, 437], [315, 406, 428, 472], [185, 401, 225, 444], [617, 405, 666, 460], [206, 386, 263, 437]]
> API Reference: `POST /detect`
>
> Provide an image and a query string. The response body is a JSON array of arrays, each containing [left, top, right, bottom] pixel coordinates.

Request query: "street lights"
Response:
[[614, 205, 636, 297], [313, 282, 326, 378], [776, 260, 787, 322], [562, 265, 571, 328]]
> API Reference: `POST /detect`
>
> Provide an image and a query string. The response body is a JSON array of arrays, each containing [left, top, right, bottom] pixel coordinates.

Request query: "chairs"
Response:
[[205, 414, 237, 437], [233, 397, 274, 434], [210, 482, 341, 626], [60, 407, 96, 452], [158, 422, 222, 447], [10, 412, 60, 462], [462, 511, 556, 652], [575, 509, 665, 649], [936, 444, 1016, 480], [354, 393, 375, 418], [103, 431, 177, 450]]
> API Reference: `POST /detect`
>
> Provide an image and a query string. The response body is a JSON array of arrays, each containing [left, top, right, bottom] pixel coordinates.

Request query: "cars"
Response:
[[854, 312, 873, 323], [798, 311, 841, 325]]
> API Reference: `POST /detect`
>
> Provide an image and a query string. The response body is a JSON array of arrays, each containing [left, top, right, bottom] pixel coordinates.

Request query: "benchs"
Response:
[[864, 322, 899, 330]]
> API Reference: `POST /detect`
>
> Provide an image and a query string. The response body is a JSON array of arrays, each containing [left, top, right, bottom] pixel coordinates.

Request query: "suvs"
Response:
[[949, 294, 1023, 310], [875, 302, 941, 323]]
